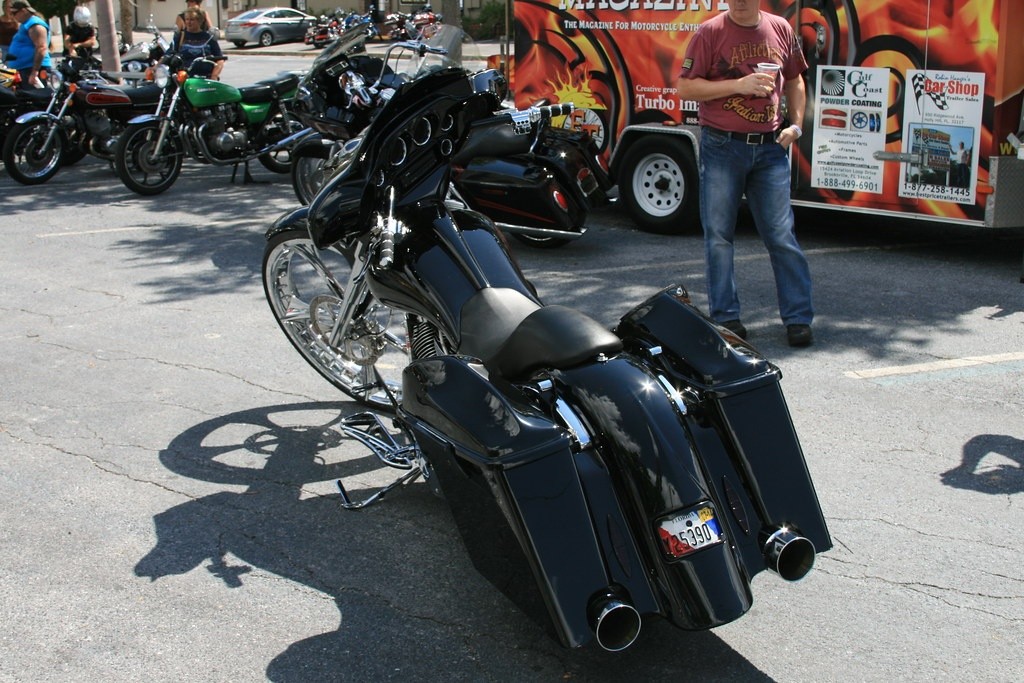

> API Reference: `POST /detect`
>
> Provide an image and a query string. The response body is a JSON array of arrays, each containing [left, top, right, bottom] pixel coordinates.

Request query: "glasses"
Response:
[[10, 10, 21, 16]]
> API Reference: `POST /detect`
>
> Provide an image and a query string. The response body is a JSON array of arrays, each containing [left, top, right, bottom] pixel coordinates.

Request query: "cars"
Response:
[[226, 7, 319, 50]]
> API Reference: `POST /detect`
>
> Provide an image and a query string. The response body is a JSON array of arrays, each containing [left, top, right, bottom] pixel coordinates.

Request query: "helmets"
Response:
[[73, 6, 91, 28]]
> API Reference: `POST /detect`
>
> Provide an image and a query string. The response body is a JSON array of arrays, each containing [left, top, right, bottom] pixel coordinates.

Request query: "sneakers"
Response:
[[722, 319, 747, 341], [787, 324, 813, 347]]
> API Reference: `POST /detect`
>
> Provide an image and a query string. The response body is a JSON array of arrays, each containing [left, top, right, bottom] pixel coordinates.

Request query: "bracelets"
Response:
[[790, 124, 802, 139]]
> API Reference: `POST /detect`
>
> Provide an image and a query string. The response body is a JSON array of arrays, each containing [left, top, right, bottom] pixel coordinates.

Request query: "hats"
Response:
[[9, 0, 36, 13]]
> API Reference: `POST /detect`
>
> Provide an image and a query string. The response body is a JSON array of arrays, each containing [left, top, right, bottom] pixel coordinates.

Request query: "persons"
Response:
[[175, 0, 223, 81], [65, 6, 96, 58], [677, 0, 815, 350], [0, 0, 51, 91], [949, 142, 972, 188]]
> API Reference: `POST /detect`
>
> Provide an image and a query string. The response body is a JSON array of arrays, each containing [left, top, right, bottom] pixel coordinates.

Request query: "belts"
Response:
[[702, 125, 782, 145]]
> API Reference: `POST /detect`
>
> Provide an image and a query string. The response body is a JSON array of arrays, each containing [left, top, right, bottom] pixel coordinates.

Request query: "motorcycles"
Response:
[[260, 26, 836, 653], [287, 24, 612, 245], [1, 5, 443, 194]]
